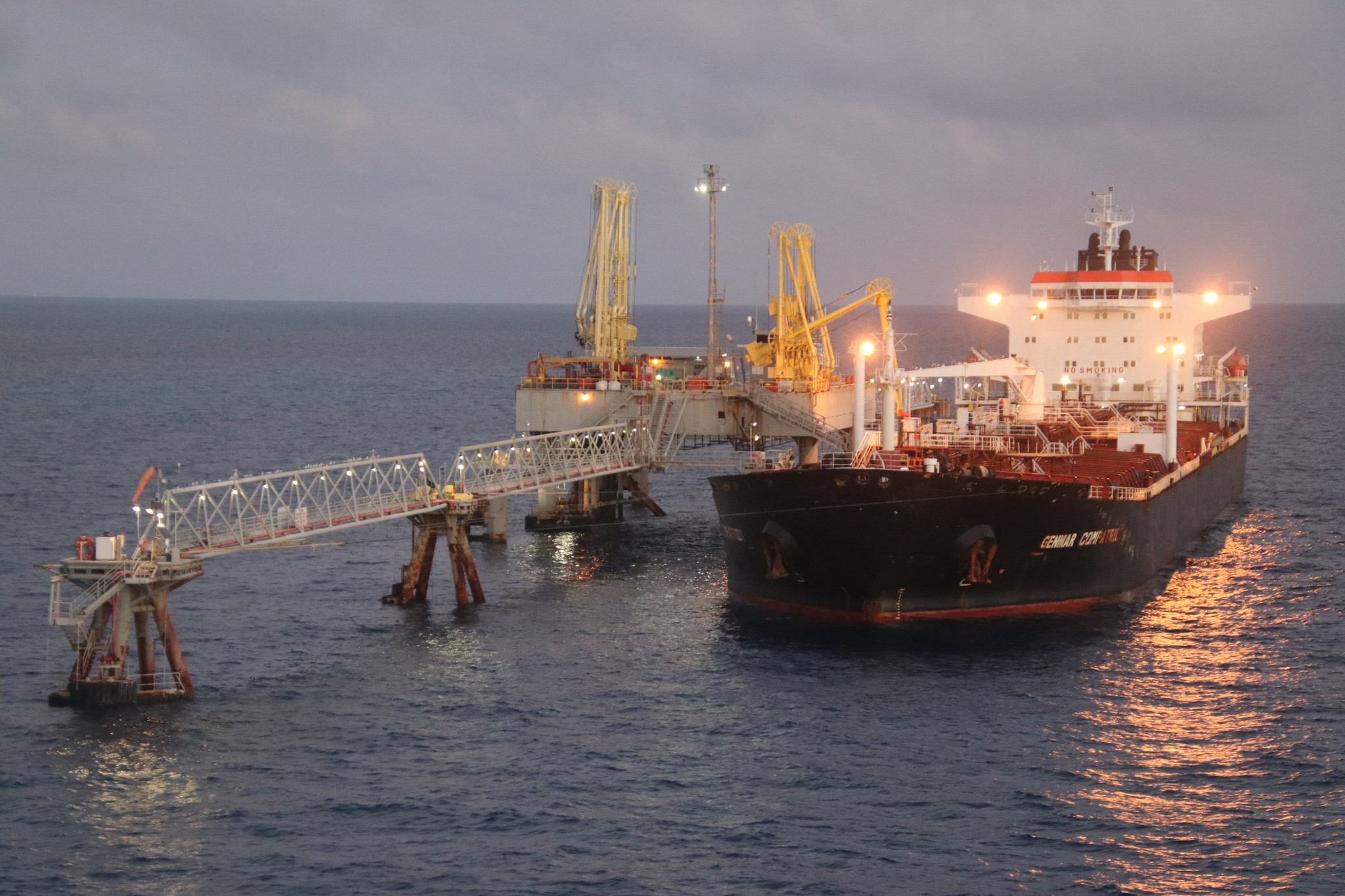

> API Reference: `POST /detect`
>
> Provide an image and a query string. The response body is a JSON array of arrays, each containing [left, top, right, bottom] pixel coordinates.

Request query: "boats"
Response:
[[1228, 364, 1248, 376], [704, 184, 1258, 648]]
